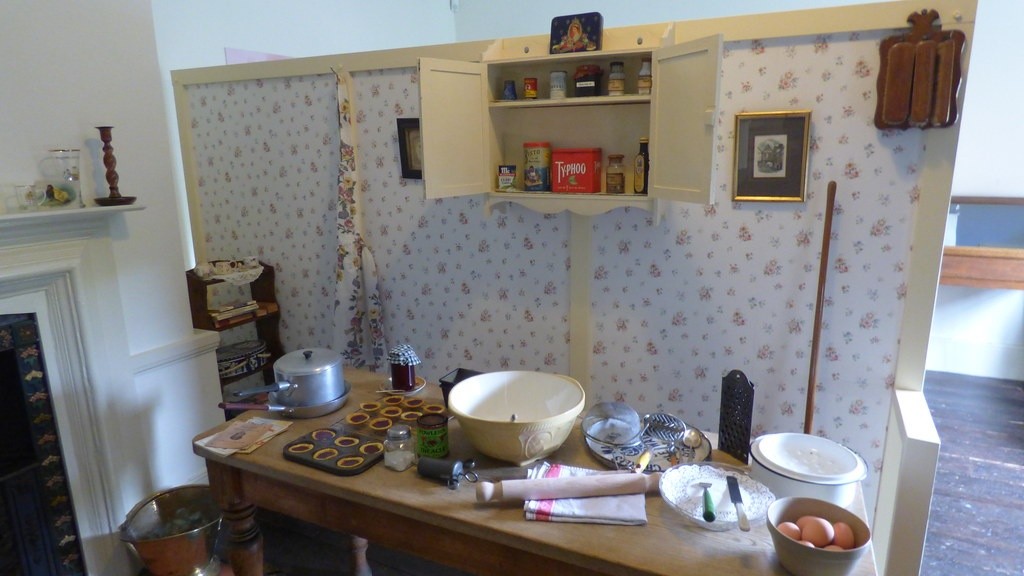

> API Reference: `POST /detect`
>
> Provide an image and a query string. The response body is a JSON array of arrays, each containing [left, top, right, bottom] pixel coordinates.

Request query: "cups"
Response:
[[49, 148, 86, 210], [215, 260, 238, 274], [15, 184, 46, 212], [243, 256, 260, 269]]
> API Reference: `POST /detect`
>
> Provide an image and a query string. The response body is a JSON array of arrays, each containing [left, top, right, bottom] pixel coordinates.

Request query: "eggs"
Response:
[[776, 515, 855, 552]]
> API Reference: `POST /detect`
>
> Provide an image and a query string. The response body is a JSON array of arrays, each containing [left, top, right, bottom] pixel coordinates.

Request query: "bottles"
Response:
[[634, 137, 649, 195], [502, 79, 517, 100], [606, 155, 626, 194], [383, 425, 417, 472], [638, 57, 652, 94], [608, 62, 626, 97], [388, 344, 415, 391]]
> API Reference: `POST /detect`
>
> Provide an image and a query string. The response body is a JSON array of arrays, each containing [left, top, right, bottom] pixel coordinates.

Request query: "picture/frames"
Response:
[[396, 117, 422, 179], [731, 110, 812, 203]]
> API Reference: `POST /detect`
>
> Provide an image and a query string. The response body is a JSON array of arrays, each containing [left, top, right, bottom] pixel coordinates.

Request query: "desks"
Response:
[[192, 367, 876, 576]]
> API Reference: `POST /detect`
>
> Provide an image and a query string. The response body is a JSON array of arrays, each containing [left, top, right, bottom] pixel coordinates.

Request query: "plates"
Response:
[[658, 461, 777, 532], [584, 413, 712, 474], [379, 376, 426, 397]]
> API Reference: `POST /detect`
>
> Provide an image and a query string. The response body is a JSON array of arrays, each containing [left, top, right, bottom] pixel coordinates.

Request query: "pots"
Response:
[[232, 347, 345, 407], [217, 379, 352, 418]]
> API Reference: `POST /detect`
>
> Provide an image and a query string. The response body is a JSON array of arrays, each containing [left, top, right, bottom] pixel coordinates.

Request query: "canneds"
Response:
[[606, 154, 625, 194], [523, 142, 551, 192], [550, 70, 567, 99], [523, 78, 537, 98], [416, 413, 448, 459], [608, 62, 624, 96]]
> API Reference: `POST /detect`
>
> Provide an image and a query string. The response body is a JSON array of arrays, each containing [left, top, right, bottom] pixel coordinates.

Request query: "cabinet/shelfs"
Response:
[[186, 258, 287, 422], [485, 47, 655, 213]]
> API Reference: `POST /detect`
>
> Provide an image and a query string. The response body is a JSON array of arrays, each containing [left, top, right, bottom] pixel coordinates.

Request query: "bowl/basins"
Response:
[[447, 369, 587, 467], [767, 496, 872, 576], [750, 431, 869, 510]]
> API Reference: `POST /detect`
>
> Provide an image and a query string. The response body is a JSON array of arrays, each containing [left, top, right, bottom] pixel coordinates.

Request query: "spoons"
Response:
[[682, 428, 701, 466]]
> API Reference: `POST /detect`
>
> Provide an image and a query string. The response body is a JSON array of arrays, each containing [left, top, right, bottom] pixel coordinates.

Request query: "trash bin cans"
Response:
[[117, 484, 221, 576]]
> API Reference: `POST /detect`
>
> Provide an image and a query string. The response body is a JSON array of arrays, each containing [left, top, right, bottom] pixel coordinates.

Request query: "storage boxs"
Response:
[[550, 148, 602, 193]]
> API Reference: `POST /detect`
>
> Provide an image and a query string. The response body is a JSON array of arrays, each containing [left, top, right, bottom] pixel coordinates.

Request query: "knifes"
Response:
[[726, 475, 750, 532]]
